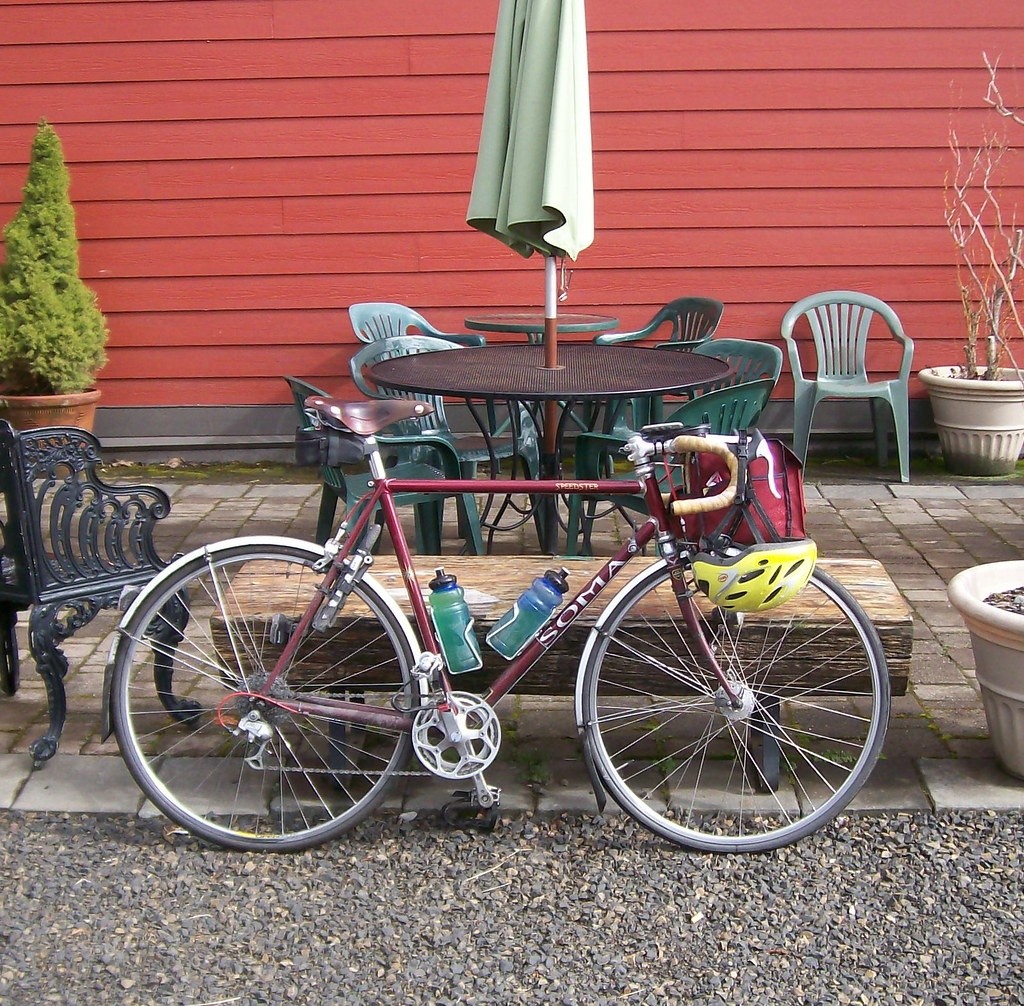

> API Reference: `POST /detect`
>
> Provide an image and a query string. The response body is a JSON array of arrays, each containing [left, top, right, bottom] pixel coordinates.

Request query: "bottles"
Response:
[[428, 567, 482, 672], [487, 567, 571, 658]]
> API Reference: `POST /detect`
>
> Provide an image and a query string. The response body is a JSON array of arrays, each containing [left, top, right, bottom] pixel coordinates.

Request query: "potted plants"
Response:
[[917, 51, 1023, 475], [0, 115, 113, 479]]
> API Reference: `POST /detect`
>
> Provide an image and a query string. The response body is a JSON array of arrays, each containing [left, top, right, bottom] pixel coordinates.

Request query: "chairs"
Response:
[[781, 290, 914, 482], [0, 419, 201, 772], [283, 375, 486, 556], [349, 303, 500, 473], [346, 335, 545, 556], [592, 296, 724, 472], [565, 338, 784, 555]]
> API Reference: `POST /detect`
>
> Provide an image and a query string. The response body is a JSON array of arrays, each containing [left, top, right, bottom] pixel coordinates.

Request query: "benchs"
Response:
[[210, 558, 912, 795]]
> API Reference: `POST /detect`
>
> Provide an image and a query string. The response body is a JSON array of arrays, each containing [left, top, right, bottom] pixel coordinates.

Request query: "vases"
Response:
[[947, 561, 1024, 782]]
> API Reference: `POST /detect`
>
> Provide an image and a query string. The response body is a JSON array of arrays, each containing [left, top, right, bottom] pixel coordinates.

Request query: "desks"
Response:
[[366, 347, 733, 556], [464, 313, 619, 343]]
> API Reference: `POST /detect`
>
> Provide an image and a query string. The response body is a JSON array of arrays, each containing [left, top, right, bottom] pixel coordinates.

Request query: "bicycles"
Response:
[[96, 394, 892, 859]]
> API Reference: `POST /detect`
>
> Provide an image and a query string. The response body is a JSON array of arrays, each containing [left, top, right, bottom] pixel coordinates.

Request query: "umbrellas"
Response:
[[467, 0, 594, 554]]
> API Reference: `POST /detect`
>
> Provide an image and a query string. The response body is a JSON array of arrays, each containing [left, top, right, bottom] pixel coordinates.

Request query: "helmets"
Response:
[[691, 538, 817, 613]]
